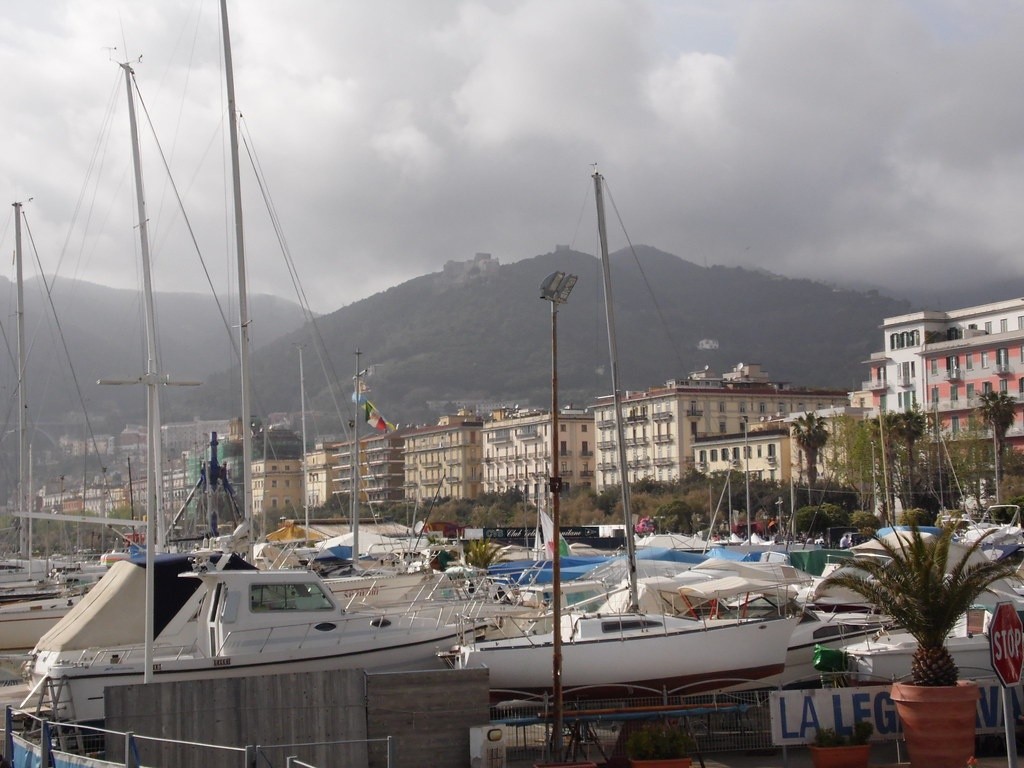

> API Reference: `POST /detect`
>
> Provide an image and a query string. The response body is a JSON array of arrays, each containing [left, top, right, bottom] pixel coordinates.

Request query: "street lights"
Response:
[[58, 474, 66, 545], [540, 271, 579, 762], [100, 467, 108, 553], [774, 497, 783, 531]]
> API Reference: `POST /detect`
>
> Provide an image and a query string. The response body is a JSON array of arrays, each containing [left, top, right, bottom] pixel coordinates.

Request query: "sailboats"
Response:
[[435, 170, 801, 689], [1, 0, 549, 721]]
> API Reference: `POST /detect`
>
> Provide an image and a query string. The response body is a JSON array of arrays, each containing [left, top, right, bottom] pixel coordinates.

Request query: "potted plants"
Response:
[[809, 719, 874, 768], [623, 726, 697, 768], [812, 506, 1024, 768]]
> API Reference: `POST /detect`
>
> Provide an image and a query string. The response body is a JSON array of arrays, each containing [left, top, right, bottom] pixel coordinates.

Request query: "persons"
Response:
[[840, 535, 851, 549]]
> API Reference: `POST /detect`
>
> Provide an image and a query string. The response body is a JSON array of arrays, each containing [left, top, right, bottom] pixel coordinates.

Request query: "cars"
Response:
[[848, 533, 869, 546], [806, 535, 828, 545]]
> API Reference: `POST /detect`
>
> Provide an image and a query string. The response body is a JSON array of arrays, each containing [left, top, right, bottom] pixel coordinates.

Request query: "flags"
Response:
[[352, 370, 398, 432], [539, 510, 576, 558]]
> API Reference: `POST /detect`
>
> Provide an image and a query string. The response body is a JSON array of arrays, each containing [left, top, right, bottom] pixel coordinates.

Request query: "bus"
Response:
[[122, 533, 145, 545]]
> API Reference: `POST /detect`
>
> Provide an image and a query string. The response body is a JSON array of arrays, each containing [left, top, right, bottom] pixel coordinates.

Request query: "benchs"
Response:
[[534, 739, 575, 761]]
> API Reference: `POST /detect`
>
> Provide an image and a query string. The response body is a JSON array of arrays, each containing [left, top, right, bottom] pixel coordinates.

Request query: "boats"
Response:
[[572, 504, 1024, 685]]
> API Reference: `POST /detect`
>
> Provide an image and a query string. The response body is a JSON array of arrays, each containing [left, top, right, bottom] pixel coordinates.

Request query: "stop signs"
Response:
[[988, 601, 1024, 688]]
